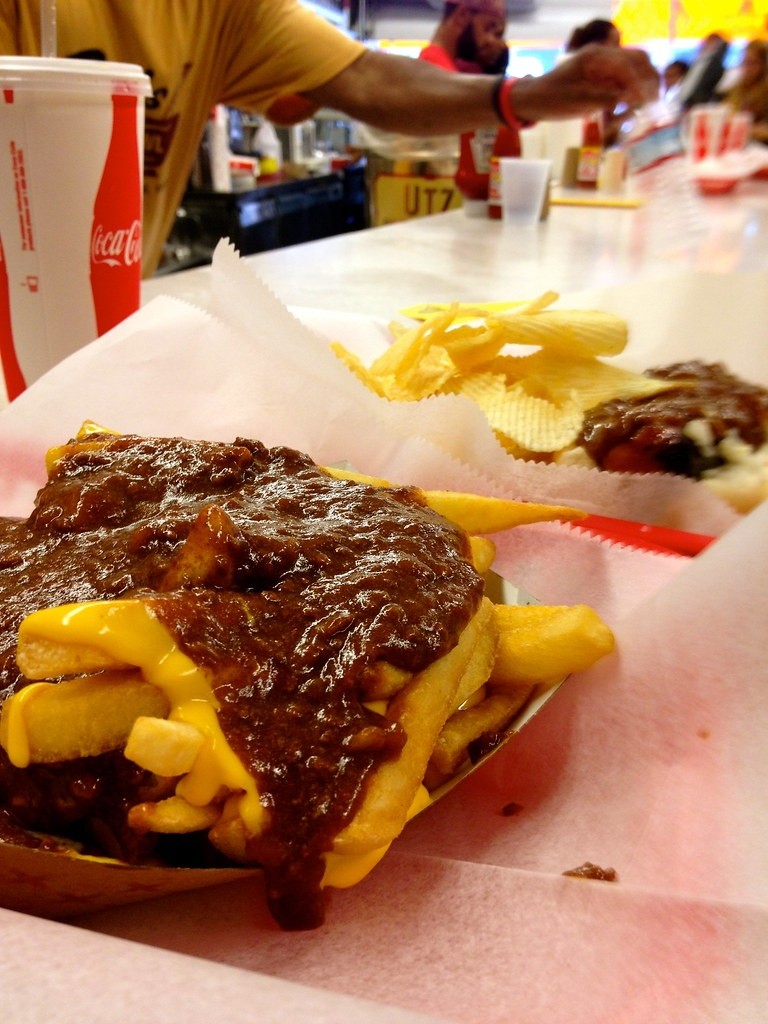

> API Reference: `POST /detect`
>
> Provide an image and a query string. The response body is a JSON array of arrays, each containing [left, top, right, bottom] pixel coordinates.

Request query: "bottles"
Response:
[[488, 126, 522, 220], [202, 103, 283, 192], [576, 107, 605, 189]]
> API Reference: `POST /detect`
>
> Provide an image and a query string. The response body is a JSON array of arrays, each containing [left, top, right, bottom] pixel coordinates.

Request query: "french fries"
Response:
[[0, 420, 616, 890]]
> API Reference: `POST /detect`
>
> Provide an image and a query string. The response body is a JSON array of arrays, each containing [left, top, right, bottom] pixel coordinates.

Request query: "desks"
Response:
[[0, 156, 768, 1024]]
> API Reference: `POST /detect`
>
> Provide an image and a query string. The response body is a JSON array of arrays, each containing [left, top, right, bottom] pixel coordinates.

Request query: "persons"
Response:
[[0, 0, 768, 277]]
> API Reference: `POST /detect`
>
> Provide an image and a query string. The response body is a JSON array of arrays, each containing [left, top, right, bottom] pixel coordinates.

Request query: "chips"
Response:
[[328, 286, 690, 452]]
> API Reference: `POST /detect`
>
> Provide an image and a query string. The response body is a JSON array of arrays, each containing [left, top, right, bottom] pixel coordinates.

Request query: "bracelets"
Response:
[[491, 76, 507, 124], [501, 77, 536, 130]]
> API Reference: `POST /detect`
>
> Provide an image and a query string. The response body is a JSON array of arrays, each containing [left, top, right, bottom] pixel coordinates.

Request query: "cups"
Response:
[[689, 110, 752, 196], [499, 158, 552, 231], [597, 152, 624, 194], [289, 120, 317, 165], [0, 56, 155, 410]]
